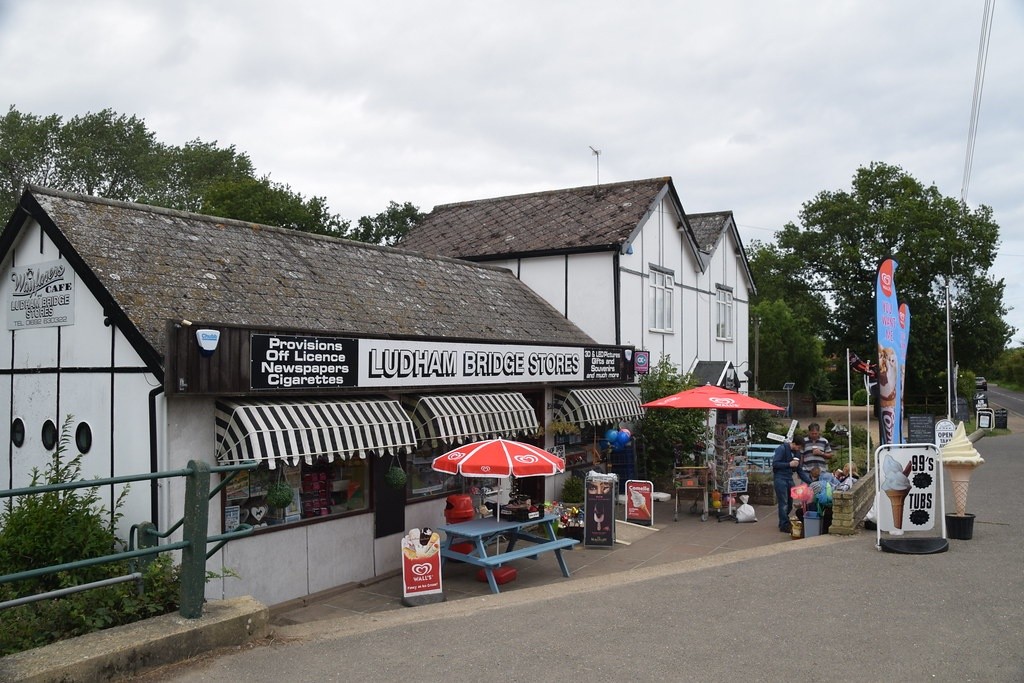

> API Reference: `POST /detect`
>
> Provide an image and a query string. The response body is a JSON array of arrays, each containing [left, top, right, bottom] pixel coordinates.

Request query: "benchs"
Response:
[[439, 528, 522, 547], [476, 538, 580, 566]]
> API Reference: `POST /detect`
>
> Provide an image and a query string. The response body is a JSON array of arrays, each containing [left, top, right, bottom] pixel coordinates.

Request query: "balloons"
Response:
[[601, 427, 632, 454]]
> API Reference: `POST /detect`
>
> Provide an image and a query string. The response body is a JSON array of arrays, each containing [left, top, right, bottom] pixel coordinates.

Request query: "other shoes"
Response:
[[780, 527, 791, 533]]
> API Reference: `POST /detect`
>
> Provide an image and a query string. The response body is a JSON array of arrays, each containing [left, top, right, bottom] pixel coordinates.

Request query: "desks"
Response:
[[436, 511, 572, 593]]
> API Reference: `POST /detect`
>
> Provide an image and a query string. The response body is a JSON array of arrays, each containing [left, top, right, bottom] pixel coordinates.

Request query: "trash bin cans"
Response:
[[995, 408, 1008, 429]]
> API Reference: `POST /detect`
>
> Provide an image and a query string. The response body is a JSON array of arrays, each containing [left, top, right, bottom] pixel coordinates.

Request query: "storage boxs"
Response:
[[304, 472, 328, 517], [558, 527, 585, 542], [500, 505, 545, 522]]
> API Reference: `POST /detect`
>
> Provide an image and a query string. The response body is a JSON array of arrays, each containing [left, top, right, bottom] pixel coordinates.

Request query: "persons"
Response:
[[589, 481, 611, 496], [772, 436, 805, 532], [794, 460, 859, 522], [799, 422, 833, 482]]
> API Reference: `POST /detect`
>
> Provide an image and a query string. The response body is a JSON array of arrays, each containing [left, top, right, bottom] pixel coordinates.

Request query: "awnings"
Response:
[[403, 392, 538, 446], [213, 400, 418, 468], [551, 389, 644, 432]]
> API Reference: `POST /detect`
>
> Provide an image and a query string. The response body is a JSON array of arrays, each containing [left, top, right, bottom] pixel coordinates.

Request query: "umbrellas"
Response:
[[431, 435, 565, 568], [638, 386, 784, 466]]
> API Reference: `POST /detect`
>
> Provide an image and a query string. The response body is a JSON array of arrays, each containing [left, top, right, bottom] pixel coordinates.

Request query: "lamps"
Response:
[[734, 360, 752, 378]]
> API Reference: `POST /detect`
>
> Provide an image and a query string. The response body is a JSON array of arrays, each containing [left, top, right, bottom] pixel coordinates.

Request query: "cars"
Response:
[[975, 377, 987, 391]]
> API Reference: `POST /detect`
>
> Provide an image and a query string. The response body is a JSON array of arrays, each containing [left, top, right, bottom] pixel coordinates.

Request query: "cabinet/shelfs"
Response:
[[673, 424, 750, 524]]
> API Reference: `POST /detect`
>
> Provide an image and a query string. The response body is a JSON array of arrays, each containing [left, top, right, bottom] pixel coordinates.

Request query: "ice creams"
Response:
[[409, 527, 439, 558], [631, 490, 650, 518], [881, 454, 913, 529], [878, 342, 898, 445], [936, 420, 986, 516]]
